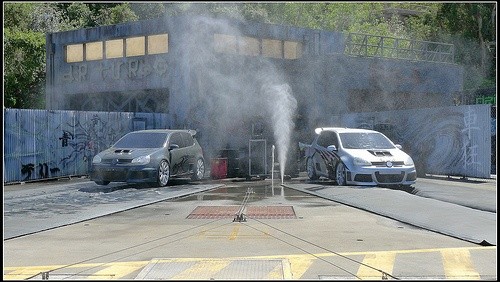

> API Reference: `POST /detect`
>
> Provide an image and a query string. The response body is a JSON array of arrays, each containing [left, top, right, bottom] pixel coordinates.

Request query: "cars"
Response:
[[89, 130, 205, 186], [305, 128, 419, 189]]
